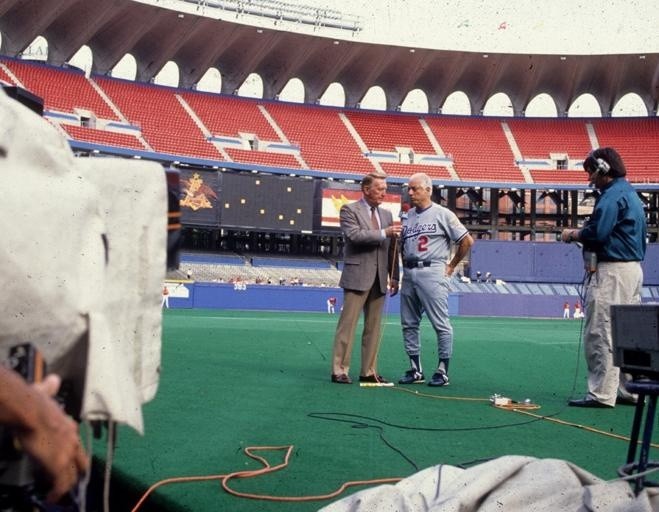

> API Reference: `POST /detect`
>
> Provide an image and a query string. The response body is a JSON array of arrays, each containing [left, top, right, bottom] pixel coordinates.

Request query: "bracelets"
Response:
[[568, 231, 574, 243]]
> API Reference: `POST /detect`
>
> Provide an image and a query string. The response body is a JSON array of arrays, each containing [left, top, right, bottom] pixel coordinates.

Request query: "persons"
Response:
[[0, 363, 89, 506], [331, 173, 403, 384], [562, 147, 647, 408], [399, 173, 473, 386], [563, 300, 581, 320], [160, 286, 169, 308]]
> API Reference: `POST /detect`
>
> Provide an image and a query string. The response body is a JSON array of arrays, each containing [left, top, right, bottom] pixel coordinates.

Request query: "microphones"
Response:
[[401, 204, 409, 227], [588, 169, 600, 188]]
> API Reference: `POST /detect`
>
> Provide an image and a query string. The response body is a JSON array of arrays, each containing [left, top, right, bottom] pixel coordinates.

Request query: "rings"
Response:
[[396, 233, 399, 236]]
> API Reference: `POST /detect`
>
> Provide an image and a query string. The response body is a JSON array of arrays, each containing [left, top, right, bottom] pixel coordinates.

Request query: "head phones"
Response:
[[589, 150, 612, 175]]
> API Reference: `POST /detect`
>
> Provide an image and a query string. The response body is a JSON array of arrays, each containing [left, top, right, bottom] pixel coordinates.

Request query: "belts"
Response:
[[403, 260, 429, 269]]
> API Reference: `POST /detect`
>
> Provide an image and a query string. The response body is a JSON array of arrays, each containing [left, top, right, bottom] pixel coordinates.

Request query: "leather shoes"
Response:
[[616, 397, 640, 405], [331, 374, 351, 383], [569, 396, 606, 408], [359, 375, 386, 383]]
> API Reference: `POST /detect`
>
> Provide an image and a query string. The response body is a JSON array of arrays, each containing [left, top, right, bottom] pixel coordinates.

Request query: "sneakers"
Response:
[[399, 371, 424, 384], [428, 374, 449, 387]]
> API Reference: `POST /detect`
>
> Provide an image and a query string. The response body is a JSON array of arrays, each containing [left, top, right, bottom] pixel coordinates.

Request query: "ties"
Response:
[[371, 206, 380, 230]]
